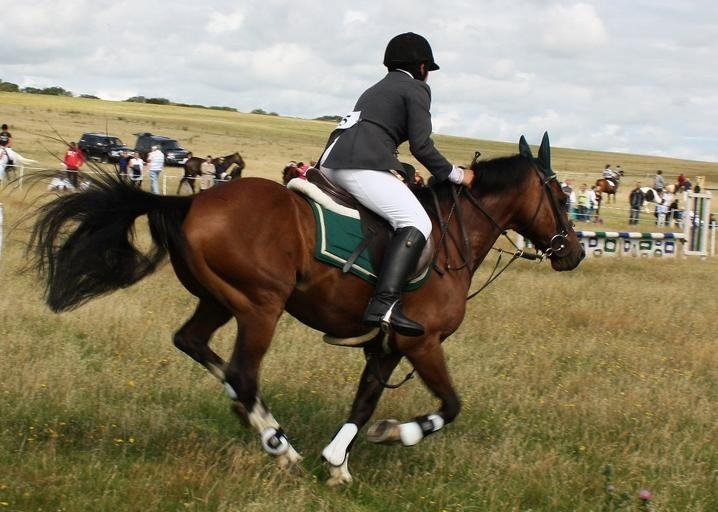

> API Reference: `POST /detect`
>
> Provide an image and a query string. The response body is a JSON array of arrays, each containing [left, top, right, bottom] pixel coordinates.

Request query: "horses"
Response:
[[4, 131, 587, 486], [628, 182, 682, 224], [595, 171, 624, 204], [282, 160, 301, 184], [677, 180, 692, 193], [177, 152, 246, 194], [0, 145, 41, 188]]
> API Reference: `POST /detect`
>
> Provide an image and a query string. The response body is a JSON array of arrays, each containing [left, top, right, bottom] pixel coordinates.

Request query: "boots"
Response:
[[363, 226, 426, 336]]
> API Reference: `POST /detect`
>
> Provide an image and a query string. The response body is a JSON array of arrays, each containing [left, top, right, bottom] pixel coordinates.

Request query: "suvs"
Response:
[[77, 131, 141, 165], [131, 133, 193, 166]]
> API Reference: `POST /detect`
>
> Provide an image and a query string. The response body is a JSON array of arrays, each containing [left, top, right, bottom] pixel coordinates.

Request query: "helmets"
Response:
[[383, 32, 440, 70]]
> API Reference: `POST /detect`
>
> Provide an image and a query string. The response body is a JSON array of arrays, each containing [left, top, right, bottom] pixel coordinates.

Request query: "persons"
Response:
[[145, 145, 165, 196], [295, 161, 306, 177], [128, 151, 144, 188], [117, 150, 129, 178], [215, 156, 233, 181], [319, 32, 476, 337], [309, 159, 317, 168], [64, 142, 83, 187], [186, 152, 193, 193], [559, 164, 716, 230], [0, 124, 12, 150], [198, 155, 216, 191]]
